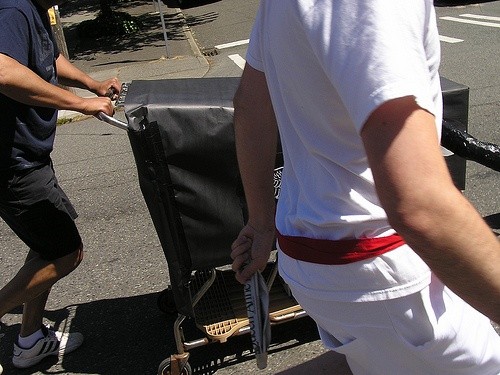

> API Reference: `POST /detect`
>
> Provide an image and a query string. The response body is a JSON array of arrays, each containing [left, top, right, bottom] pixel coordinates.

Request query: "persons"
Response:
[[0, 0, 121, 375], [230, 0, 500, 375]]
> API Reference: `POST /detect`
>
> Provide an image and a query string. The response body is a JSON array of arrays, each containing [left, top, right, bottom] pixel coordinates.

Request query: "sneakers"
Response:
[[12, 330, 84, 368]]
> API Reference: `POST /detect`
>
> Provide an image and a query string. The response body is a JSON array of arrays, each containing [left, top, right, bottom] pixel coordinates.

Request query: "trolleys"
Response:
[[97, 77, 470, 374]]
